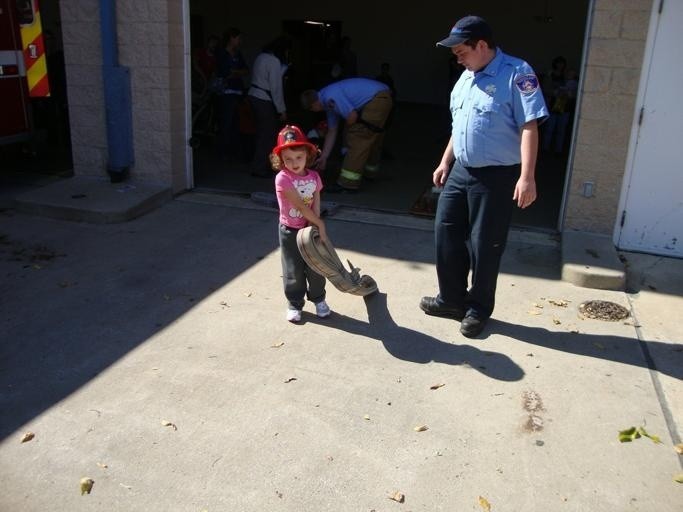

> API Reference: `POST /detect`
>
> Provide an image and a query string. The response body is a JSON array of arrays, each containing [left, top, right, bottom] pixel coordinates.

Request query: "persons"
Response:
[[420, 14, 549, 336], [542, 53, 572, 158], [269, 122, 333, 323], [218, 29, 248, 159], [300, 76, 392, 196], [246, 40, 290, 178], [328, 36, 358, 80], [367, 62, 396, 110]]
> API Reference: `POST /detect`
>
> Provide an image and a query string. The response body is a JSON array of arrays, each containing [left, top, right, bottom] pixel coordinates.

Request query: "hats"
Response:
[[273, 126, 318, 161], [436, 14, 495, 48]]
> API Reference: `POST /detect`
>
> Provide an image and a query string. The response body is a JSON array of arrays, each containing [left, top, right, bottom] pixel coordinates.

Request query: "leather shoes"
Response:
[[419, 297, 465, 318], [322, 183, 357, 194], [460, 315, 484, 337]]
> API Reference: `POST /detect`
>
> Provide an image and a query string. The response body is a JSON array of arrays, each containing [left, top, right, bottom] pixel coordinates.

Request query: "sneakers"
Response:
[[250, 168, 271, 178], [287, 310, 302, 322], [315, 300, 331, 319]]
